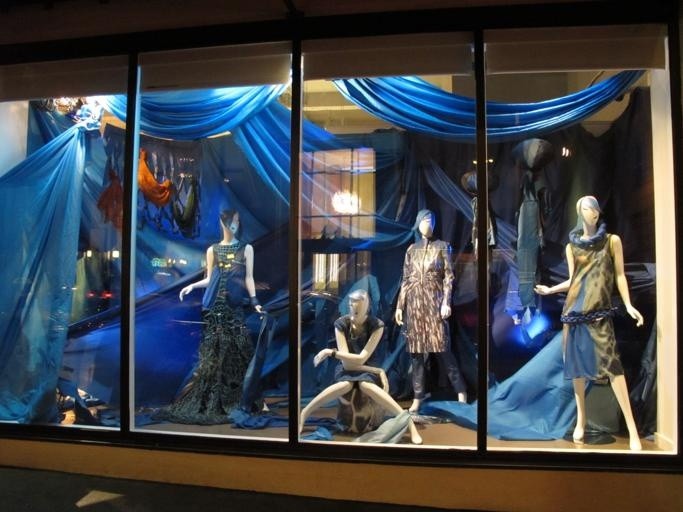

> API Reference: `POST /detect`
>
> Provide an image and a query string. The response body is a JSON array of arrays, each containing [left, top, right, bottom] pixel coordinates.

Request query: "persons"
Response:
[[390, 208, 469, 414], [529, 194, 647, 450], [151, 208, 268, 421], [292, 288, 423, 446]]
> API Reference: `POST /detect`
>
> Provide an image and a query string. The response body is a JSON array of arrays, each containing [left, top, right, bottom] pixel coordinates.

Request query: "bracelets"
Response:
[[331, 348, 336, 357], [249, 297, 260, 307]]
